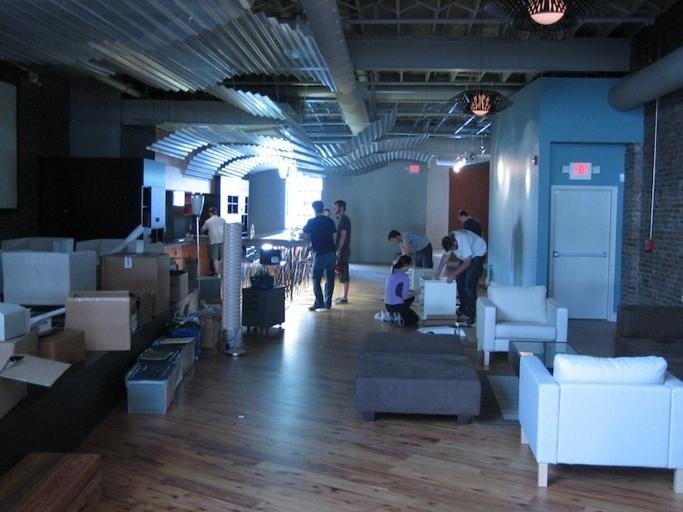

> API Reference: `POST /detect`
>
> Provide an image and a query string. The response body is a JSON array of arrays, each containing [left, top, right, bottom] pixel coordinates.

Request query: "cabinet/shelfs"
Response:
[[241, 286, 287, 336], [390, 261, 434, 290], [418, 275, 457, 321], [142, 185, 166, 229]]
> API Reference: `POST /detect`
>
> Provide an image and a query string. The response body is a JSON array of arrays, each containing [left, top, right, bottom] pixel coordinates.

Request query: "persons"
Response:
[[433, 229, 488, 322], [302, 200, 336, 311], [387, 228, 434, 268], [372, 254, 419, 326], [331, 201, 352, 304], [457, 210, 483, 280], [321, 208, 330, 216], [197, 206, 226, 276]]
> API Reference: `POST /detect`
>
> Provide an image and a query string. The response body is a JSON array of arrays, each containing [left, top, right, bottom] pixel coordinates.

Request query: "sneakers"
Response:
[[333, 297, 348, 305], [308, 301, 331, 312], [457, 316, 475, 327]]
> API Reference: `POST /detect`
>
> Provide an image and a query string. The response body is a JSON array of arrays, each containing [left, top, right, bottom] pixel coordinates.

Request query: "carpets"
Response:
[[487, 374, 519, 421]]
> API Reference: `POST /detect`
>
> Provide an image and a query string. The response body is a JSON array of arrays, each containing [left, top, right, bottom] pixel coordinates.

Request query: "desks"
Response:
[[507, 339, 582, 379]]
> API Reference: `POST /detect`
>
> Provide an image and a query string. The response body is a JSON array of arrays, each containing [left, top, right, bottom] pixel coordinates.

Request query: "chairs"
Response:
[[476, 296, 569, 366], [241, 228, 314, 300], [518, 355, 683, 496]]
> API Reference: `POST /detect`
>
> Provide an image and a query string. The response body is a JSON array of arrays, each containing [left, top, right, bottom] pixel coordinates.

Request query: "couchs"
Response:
[[616, 303, 683, 382]]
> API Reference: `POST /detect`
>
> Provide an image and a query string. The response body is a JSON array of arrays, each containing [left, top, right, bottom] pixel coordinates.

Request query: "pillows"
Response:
[[487, 280, 548, 323], [552, 353, 667, 383]]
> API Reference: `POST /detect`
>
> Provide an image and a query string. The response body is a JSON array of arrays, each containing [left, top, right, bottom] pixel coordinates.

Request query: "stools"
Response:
[[355, 330, 483, 425]]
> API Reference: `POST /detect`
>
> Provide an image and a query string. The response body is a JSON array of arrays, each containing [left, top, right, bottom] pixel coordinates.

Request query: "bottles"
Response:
[[249, 223, 255, 238]]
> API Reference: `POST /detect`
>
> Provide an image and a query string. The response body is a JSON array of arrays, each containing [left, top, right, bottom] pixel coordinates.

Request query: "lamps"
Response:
[[189, 193, 205, 313], [482, 0, 611, 35], [447, 5, 514, 116]]
